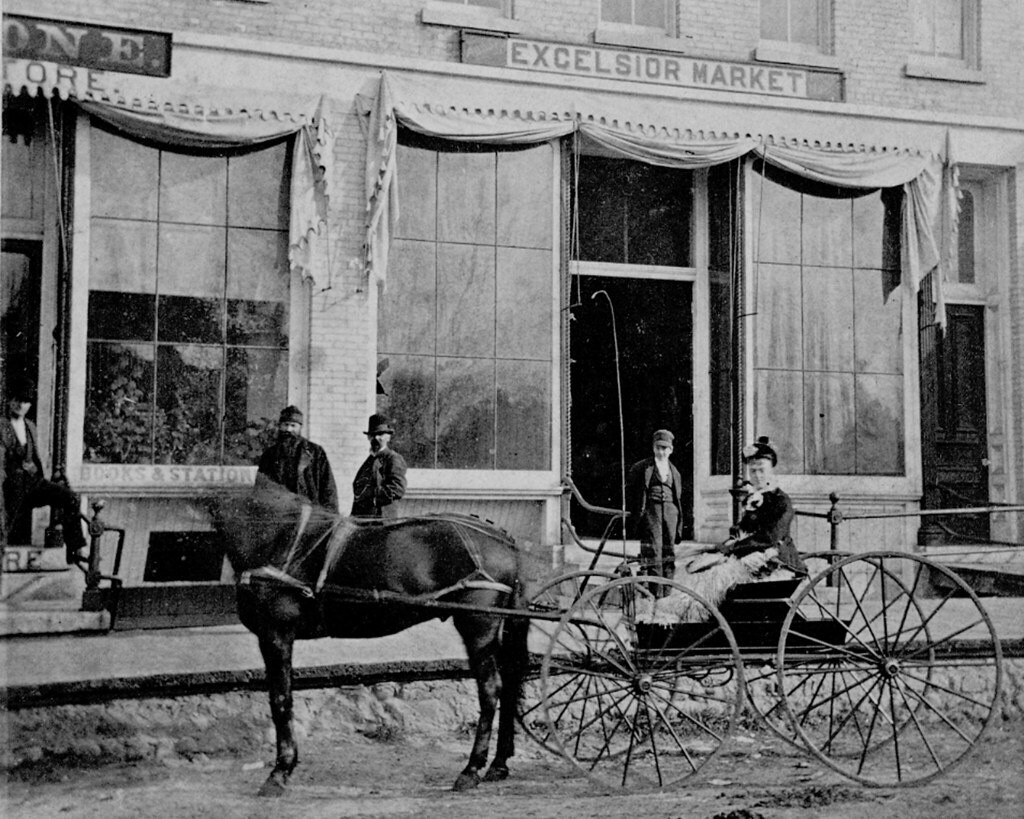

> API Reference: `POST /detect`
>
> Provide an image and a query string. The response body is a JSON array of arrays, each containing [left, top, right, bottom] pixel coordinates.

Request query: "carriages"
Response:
[[122, 432, 1004, 801]]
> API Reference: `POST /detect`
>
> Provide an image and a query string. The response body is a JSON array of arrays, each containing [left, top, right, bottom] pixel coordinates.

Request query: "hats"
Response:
[[363, 414, 394, 435], [741, 436, 777, 467], [6, 377, 35, 403], [653, 430, 674, 446], [279, 406, 303, 425]]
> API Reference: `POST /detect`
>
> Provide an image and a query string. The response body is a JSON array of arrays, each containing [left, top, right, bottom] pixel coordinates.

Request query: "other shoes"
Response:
[[67, 547, 80, 562]]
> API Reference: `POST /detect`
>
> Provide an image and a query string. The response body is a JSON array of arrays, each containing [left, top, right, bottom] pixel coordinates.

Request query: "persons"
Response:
[[349, 414, 407, 525], [625, 430, 682, 597], [643, 436, 812, 623], [0, 377, 101, 565], [256, 405, 338, 512]]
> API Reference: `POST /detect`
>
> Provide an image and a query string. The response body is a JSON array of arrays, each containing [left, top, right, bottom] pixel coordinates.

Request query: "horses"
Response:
[[168, 422, 532, 796]]
